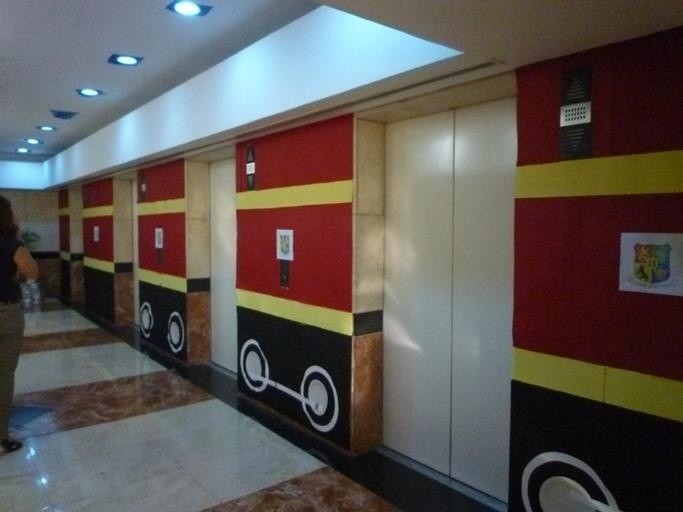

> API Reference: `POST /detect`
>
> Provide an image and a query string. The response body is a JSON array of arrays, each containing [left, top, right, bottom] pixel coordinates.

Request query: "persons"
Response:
[[0, 197, 40, 455]]
